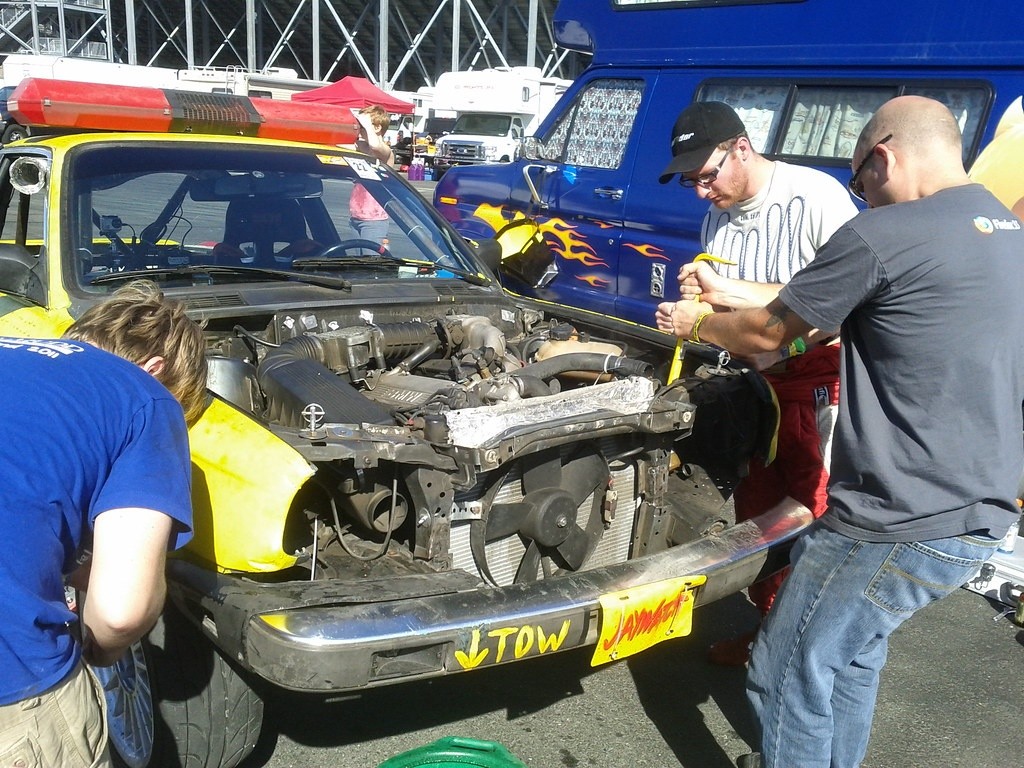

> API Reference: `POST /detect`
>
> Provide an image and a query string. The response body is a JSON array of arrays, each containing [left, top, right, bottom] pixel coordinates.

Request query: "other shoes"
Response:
[[707, 632, 753, 664], [742, 751, 763, 767]]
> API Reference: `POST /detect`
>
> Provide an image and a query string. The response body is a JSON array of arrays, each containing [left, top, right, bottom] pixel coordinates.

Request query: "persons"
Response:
[[347, 103, 394, 256], [658, 100, 861, 670], [1, 275, 217, 768], [737, 94, 1022, 767], [397, 117, 414, 166]]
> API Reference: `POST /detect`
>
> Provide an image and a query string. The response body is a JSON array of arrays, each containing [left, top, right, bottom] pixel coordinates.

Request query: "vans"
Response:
[[0, 54, 574, 186], [430, 2, 1023, 625]]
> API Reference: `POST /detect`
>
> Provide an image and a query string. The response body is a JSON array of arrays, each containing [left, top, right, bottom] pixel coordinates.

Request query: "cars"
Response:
[[0, 74, 815, 768]]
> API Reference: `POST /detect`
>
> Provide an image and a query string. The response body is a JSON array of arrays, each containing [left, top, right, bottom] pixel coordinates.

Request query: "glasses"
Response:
[[848, 135, 894, 203], [679, 148, 729, 188]]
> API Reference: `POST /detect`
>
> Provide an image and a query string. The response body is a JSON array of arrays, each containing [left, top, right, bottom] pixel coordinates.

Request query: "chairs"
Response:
[[212, 194, 332, 265]]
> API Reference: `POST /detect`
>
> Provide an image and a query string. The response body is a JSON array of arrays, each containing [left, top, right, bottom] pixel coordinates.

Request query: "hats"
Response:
[[658, 99, 746, 186]]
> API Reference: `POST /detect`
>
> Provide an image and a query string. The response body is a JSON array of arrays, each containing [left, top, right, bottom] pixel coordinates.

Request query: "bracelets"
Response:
[[780, 345, 790, 359], [693, 311, 716, 343], [787, 341, 797, 357], [793, 336, 806, 354]]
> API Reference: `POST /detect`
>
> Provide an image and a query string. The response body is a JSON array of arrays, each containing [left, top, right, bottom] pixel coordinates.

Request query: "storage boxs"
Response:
[[394, 136, 439, 181]]
